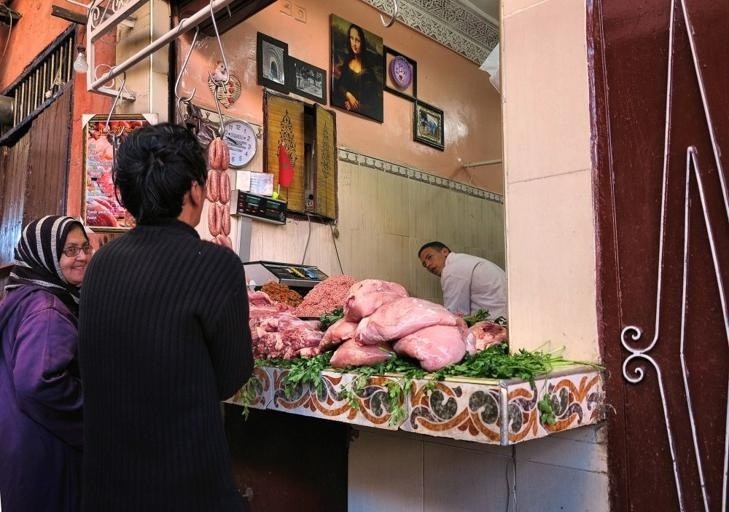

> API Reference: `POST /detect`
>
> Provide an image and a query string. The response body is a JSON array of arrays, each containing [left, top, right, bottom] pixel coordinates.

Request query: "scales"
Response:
[[229, 189, 329, 286]]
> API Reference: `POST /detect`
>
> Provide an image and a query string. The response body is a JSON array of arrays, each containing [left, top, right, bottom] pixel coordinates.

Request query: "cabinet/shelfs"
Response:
[[263, 91, 338, 223]]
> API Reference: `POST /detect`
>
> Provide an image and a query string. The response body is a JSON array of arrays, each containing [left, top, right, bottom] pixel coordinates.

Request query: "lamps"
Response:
[[71, 0, 102, 73]]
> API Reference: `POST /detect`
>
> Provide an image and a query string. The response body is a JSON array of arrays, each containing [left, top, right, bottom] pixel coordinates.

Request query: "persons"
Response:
[[417, 241, 507, 325], [73, 120, 254, 510], [334, 24, 381, 117], [1, 213, 98, 511]]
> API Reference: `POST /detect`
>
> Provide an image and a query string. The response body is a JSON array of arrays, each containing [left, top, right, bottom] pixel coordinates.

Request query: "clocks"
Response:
[[224, 120, 257, 169]]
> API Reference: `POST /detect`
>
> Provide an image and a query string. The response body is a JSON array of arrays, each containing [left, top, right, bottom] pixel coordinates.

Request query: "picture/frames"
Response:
[[328, 12, 384, 123], [77, 112, 159, 234], [413, 98, 445, 151], [289, 55, 327, 105], [383, 45, 417, 102], [256, 31, 289, 94]]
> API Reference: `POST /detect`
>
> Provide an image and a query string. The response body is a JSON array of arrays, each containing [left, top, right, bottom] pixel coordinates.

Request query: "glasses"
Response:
[[62, 244, 94, 259]]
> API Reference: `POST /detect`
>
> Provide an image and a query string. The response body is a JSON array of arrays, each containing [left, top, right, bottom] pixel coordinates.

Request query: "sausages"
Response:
[[205, 136, 232, 248]]
